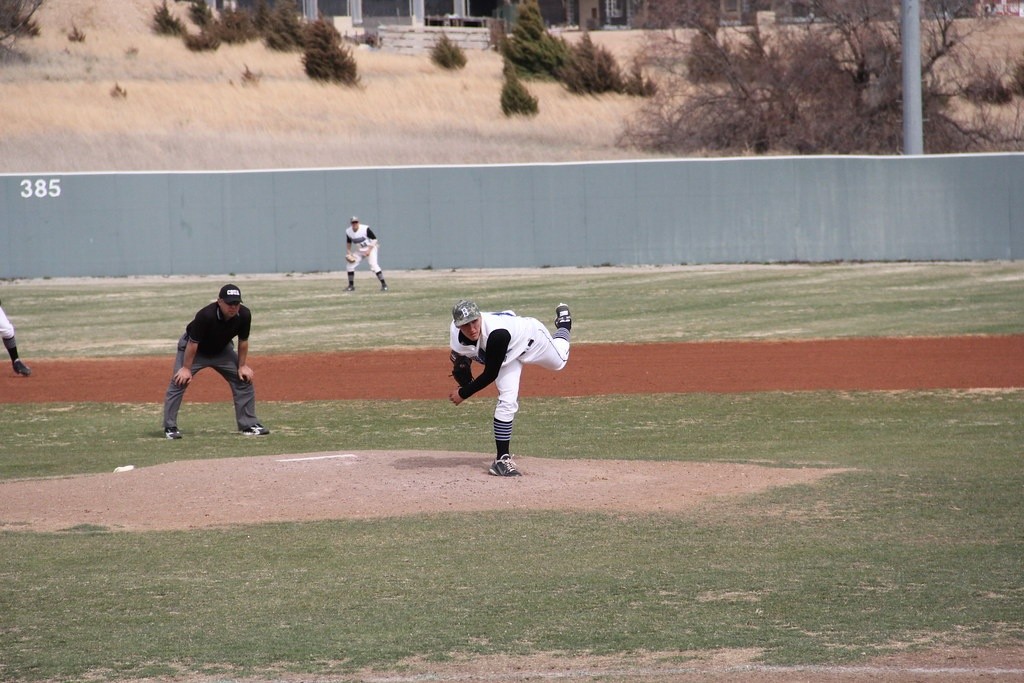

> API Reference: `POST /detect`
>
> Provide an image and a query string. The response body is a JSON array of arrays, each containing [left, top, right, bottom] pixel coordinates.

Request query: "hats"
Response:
[[452, 299, 481, 327], [350, 216, 359, 223], [219, 284, 243, 304]]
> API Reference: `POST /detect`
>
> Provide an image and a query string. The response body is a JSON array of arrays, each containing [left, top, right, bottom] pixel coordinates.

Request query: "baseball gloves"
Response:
[[448, 355, 475, 387], [345, 254, 355, 263]]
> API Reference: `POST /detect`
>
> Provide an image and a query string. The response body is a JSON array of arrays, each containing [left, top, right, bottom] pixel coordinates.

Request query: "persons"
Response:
[[163, 284, 269, 439], [0, 300, 30, 376], [449, 300, 572, 477], [345, 216, 387, 291]]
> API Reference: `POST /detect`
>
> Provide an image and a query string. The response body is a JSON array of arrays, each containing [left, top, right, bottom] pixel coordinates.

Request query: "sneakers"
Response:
[[380, 285, 388, 291], [554, 303, 572, 329], [239, 423, 270, 435], [164, 427, 182, 439], [13, 361, 31, 376], [343, 285, 355, 292], [489, 455, 521, 477]]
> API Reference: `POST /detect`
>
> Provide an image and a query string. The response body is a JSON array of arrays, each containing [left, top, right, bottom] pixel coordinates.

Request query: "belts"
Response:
[[520, 339, 534, 356]]
